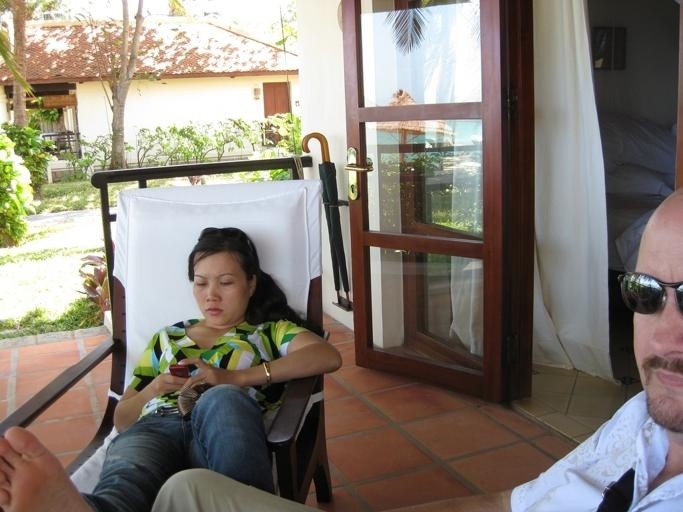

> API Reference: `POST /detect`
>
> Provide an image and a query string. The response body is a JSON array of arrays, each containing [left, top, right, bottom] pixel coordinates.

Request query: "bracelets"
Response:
[[262, 359, 274, 387]]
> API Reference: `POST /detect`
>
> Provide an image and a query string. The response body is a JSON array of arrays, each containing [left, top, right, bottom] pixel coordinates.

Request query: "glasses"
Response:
[[616, 272, 682, 316]]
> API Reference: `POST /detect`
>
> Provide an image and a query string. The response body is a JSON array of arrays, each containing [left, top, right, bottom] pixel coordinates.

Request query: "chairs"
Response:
[[1, 155, 329, 511]]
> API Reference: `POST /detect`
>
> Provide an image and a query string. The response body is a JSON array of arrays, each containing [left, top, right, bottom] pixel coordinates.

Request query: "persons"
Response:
[[0, 225, 344, 512], [148, 185, 683, 511]]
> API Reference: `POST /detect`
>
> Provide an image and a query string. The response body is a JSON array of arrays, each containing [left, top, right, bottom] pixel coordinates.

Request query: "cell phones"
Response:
[[169, 365, 190, 378]]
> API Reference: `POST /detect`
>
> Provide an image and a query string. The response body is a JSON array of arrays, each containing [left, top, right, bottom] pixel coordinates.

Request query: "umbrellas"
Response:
[[301, 134, 351, 306], [300, 131, 339, 308]]
